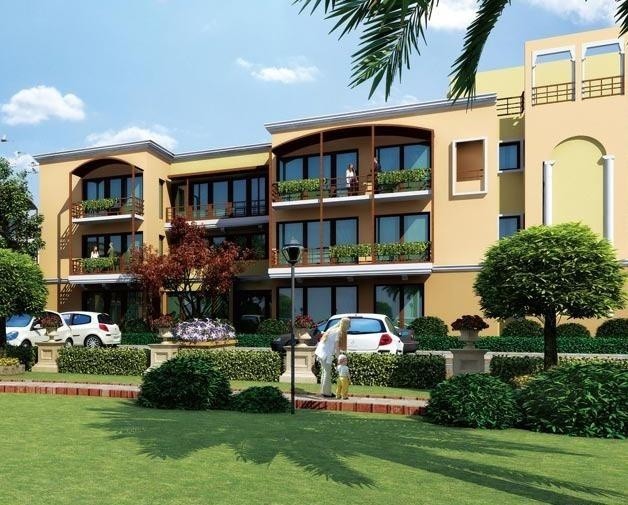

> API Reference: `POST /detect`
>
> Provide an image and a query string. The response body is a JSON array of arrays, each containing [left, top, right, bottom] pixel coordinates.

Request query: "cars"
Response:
[[269, 318, 417, 353], [315, 313, 405, 358], [5, 309, 122, 350]]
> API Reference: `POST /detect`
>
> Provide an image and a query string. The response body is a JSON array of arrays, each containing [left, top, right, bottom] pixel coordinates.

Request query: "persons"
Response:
[[335, 354, 352, 400], [107, 242, 114, 258], [346, 164, 359, 196], [91, 245, 100, 260], [370, 150, 378, 193], [314, 318, 351, 398]]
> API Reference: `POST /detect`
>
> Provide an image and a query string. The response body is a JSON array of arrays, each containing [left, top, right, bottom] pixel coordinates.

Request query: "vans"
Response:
[[239, 313, 264, 325]]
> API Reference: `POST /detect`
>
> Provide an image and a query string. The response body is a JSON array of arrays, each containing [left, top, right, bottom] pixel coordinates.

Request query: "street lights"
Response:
[[278, 236, 308, 415]]
